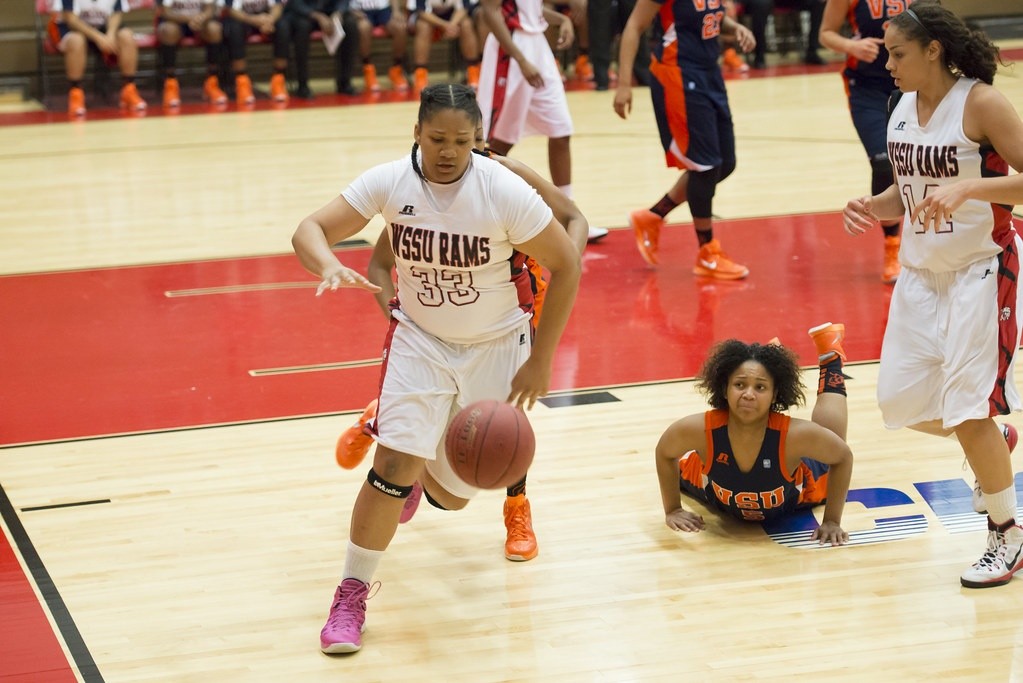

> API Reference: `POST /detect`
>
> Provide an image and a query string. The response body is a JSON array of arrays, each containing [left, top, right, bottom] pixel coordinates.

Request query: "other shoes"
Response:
[[575, 55, 594, 81], [299, 85, 310, 98], [753, 61, 766, 70], [596, 84, 608, 90], [804, 49, 827, 65], [338, 82, 355, 94]]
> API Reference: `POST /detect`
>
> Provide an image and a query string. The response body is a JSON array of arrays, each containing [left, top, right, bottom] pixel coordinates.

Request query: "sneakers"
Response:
[[692, 239, 749, 280], [588, 226, 609, 239], [414, 67, 429, 91], [161, 77, 181, 107], [960, 527, 1023, 587], [235, 74, 256, 104], [467, 64, 480, 89], [881, 235, 900, 283], [202, 75, 229, 104], [69, 88, 86, 115], [119, 83, 147, 110], [389, 64, 410, 90], [398, 481, 422, 523], [364, 65, 380, 91], [629, 210, 664, 266], [269, 72, 289, 102], [767, 337, 781, 348], [723, 48, 751, 71], [320, 578, 369, 654], [502, 499, 538, 561], [971, 422, 1018, 514], [808, 321, 846, 366], [335, 397, 379, 470]]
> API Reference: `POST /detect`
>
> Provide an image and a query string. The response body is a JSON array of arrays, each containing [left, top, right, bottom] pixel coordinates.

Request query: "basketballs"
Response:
[[444, 400, 535, 490]]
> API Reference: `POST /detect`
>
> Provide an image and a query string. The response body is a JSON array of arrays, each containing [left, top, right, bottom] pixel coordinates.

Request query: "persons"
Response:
[[289, 82, 582, 655], [656, 322, 854, 545], [337, 107, 588, 561], [33, 0, 937, 285], [844, 9, 1023, 588]]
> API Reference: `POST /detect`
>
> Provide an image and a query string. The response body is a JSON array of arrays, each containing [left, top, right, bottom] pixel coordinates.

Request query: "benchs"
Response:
[[22, 1, 854, 116]]
